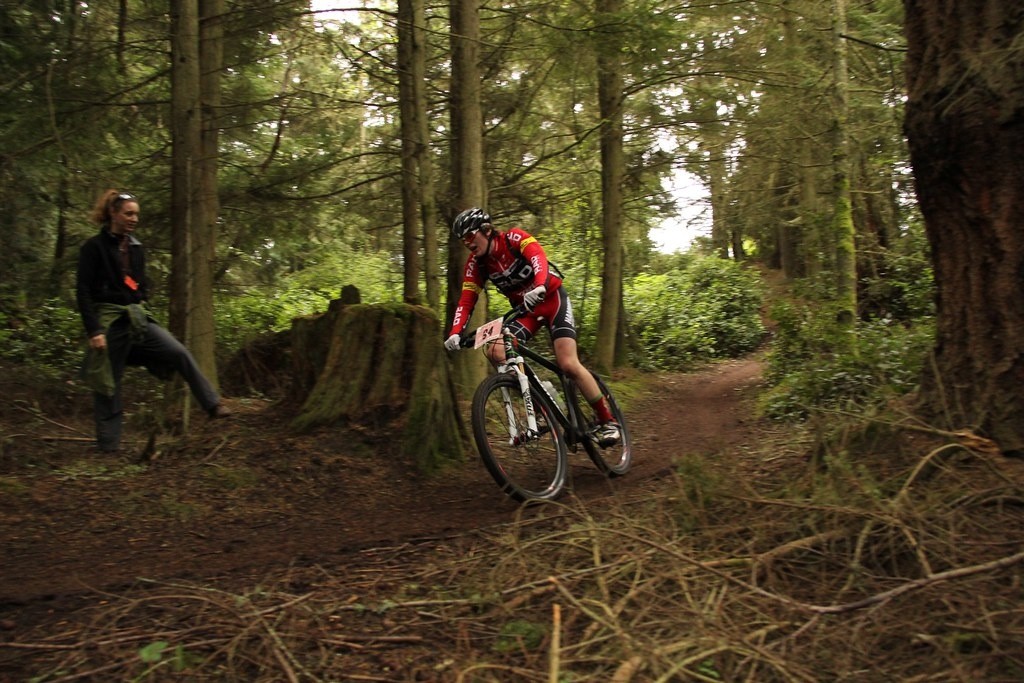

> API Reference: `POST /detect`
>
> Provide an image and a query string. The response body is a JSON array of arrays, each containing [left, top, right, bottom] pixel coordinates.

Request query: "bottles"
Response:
[[542, 381, 566, 411]]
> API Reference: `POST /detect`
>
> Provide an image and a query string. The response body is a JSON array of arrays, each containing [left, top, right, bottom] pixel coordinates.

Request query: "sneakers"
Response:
[[595, 422, 624, 449]]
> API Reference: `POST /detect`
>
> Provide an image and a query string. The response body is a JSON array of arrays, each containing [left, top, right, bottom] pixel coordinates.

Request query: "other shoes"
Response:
[[211, 407, 236, 420]]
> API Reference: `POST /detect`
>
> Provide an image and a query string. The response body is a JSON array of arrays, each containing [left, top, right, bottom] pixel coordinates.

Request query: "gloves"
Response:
[[523, 285, 547, 313], [443, 334, 463, 352]]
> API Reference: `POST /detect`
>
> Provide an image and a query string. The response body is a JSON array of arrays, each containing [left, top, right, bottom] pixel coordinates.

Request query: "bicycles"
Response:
[[444, 291, 631, 505]]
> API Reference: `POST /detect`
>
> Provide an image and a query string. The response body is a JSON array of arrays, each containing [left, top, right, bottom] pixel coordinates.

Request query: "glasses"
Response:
[[113, 191, 141, 204], [462, 229, 480, 242]]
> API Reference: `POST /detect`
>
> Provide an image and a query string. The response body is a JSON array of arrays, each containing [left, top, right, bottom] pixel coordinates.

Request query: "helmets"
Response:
[[452, 207, 494, 240]]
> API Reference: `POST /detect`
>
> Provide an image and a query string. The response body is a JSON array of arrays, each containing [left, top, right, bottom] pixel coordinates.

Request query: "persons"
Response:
[[444, 207, 622, 446], [77, 191, 232, 453]]
